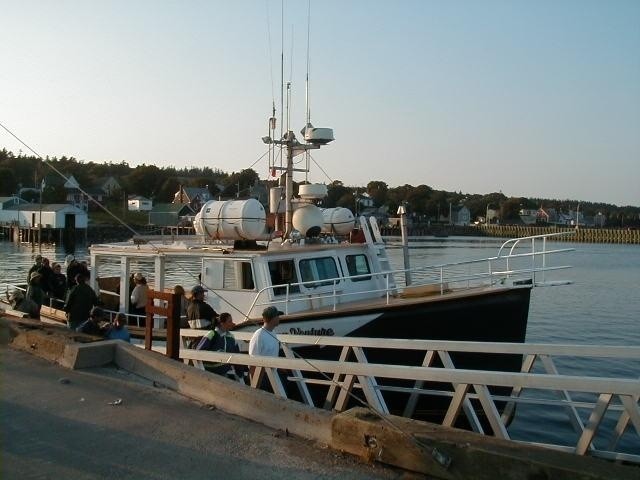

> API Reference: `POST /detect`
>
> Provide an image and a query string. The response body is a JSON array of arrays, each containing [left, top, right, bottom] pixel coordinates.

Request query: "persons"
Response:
[[26, 254, 105, 328], [194, 313, 245, 383], [76, 307, 111, 336], [248, 306, 290, 399], [9, 288, 38, 318], [115, 273, 135, 318], [131, 272, 149, 327], [174, 285, 189, 327], [187, 287, 218, 350], [103, 314, 130, 342]]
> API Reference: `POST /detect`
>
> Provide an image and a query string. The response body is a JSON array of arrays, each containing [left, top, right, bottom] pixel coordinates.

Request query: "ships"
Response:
[[0, 7, 578, 435]]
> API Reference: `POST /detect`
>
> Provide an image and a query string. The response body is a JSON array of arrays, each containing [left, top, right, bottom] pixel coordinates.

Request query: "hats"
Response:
[[66, 255, 75, 262], [263, 306, 284, 320], [29, 272, 41, 282], [134, 273, 143, 280], [35, 255, 43, 262], [90, 307, 105, 316], [52, 262, 59, 270], [9, 290, 22, 301], [190, 285, 208, 295]]
